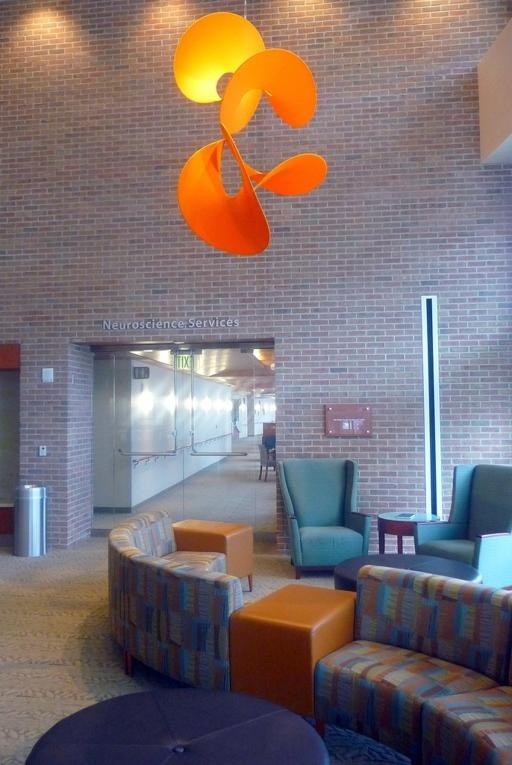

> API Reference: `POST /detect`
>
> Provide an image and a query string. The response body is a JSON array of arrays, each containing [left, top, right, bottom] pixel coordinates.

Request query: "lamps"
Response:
[[169, 0, 329, 262]]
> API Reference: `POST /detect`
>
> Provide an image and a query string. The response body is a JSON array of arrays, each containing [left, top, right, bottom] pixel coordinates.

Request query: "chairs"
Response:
[[258, 443, 275, 483], [277, 458, 511, 589]]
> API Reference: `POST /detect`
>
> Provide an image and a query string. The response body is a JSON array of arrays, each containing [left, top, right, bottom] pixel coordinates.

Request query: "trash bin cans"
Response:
[[14, 484, 47, 557]]
